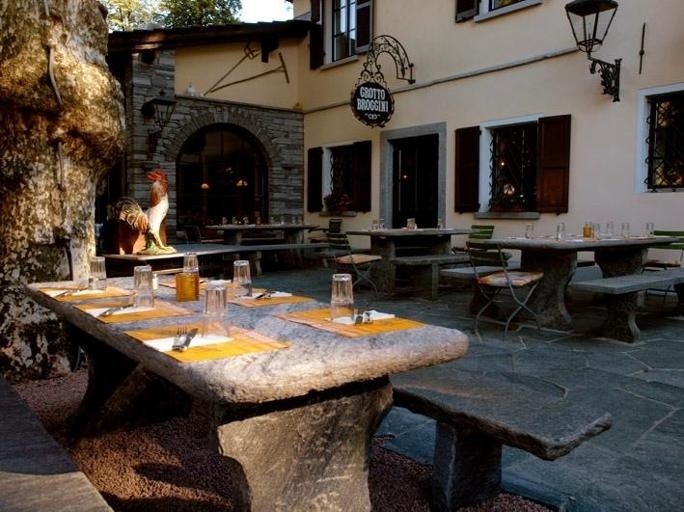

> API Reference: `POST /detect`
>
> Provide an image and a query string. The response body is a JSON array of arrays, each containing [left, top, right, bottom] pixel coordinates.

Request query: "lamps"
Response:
[[565, 0, 622, 105], [140, 88, 178, 132]]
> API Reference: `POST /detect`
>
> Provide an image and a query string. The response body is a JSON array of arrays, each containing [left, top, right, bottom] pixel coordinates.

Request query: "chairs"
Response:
[[182, 224, 224, 245], [644, 230, 683, 305]]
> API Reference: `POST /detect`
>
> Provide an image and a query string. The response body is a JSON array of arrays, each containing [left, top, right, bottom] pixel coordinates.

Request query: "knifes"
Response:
[[355, 308, 364, 327], [172, 329, 202, 353]]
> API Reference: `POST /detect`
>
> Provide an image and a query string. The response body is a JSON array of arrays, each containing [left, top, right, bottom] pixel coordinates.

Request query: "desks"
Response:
[[101, 241, 241, 276], [203, 218, 679, 332]]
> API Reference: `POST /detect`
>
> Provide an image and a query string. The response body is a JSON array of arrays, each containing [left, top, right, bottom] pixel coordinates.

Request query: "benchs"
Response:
[[572, 268, 684, 343], [392, 363, 612, 512]]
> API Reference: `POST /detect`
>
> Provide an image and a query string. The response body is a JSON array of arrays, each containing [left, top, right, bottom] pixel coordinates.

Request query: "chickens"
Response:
[[110, 168, 174, 253]]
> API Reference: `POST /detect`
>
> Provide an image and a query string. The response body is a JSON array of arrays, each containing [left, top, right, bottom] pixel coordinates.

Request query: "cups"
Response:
[[524, 221, 655, 241], [87, 253, 354, 321], [406, 219, 416, 230], [372, 219, 385, 230]]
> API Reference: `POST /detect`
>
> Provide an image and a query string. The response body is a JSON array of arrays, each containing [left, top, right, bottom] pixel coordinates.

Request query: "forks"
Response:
[[174, 324, 187, 351]]
